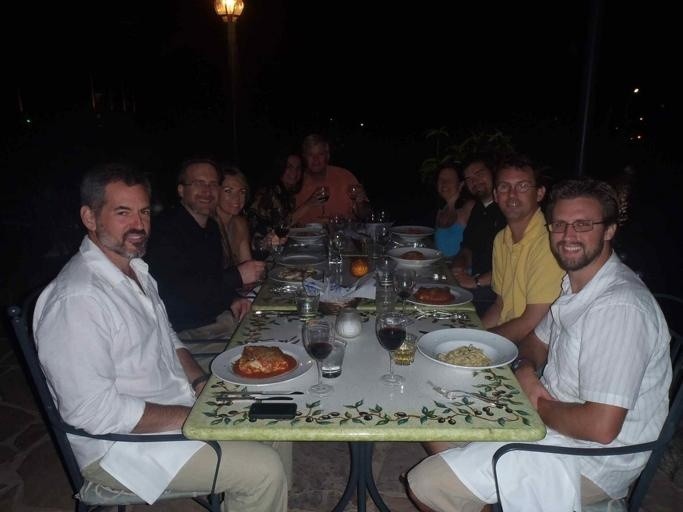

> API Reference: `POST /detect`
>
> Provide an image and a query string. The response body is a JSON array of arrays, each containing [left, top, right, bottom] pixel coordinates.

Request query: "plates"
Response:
[[415, 327, 519, 371], [285, 226, 326, 240], [209, 341, 317, 387], [266, 265, 330, 286], [404, 282, 474, 307], [273, 249, 326, 267], [387, 225, 435, 243], [385, 246, 443, 268]]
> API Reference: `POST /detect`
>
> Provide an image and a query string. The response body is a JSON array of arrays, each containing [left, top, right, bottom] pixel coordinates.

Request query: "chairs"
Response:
[[490, 293, 682, 512], [62, 238, 231, 346], [9, 281, 222, 512]]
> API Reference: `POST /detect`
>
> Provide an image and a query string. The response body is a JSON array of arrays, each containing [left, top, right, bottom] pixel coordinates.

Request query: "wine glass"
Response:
[[347, 183, 362, 213], [375, 311, 408, 387], [392, 269, 416, 327], [315, 185, 331, 219], [272, 214, 292, 256], [376, 260, 396, 304], [376, 224, 390, 257], [302, 318, 335, 394]]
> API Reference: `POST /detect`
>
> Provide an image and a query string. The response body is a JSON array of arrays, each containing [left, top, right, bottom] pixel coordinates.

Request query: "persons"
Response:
[[31, 162, 289, 512], [405, 176, 674, 511], [433, 138, 567, 369], [143, 132, 373, 370]]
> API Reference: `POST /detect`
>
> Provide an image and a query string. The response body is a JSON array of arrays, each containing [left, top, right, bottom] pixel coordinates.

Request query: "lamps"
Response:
[[212, 0, 246, 25]]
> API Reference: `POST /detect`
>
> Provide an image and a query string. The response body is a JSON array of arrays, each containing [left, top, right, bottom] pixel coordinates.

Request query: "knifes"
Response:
[[214, 395, 295, 402], [211, 389, 306, 397]]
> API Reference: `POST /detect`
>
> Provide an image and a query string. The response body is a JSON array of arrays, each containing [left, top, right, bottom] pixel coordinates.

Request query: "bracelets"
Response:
[[192, 374, 211, 388]]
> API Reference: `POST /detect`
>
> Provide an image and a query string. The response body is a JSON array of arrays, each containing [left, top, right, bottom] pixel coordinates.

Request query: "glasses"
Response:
[[545, 219, 612, 233], [182, 180, 219, 189], [496, 182, 538, 193]]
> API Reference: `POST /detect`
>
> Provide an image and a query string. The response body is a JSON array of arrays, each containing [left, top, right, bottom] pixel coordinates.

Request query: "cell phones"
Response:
[[248, 402, 297, 420]]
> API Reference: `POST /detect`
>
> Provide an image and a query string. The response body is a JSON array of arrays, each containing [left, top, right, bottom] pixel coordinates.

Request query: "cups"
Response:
[[376, 286, 395, 314], [319, 337, 346, 379], [390, 327, 419, 366], [335, 305, 363, 337], [293, 286, 319, 322]]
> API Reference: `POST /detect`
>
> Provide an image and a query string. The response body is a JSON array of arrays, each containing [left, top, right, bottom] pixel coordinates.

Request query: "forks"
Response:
[[241, 335, 301, 344], [428, 381, 499, 407]]
